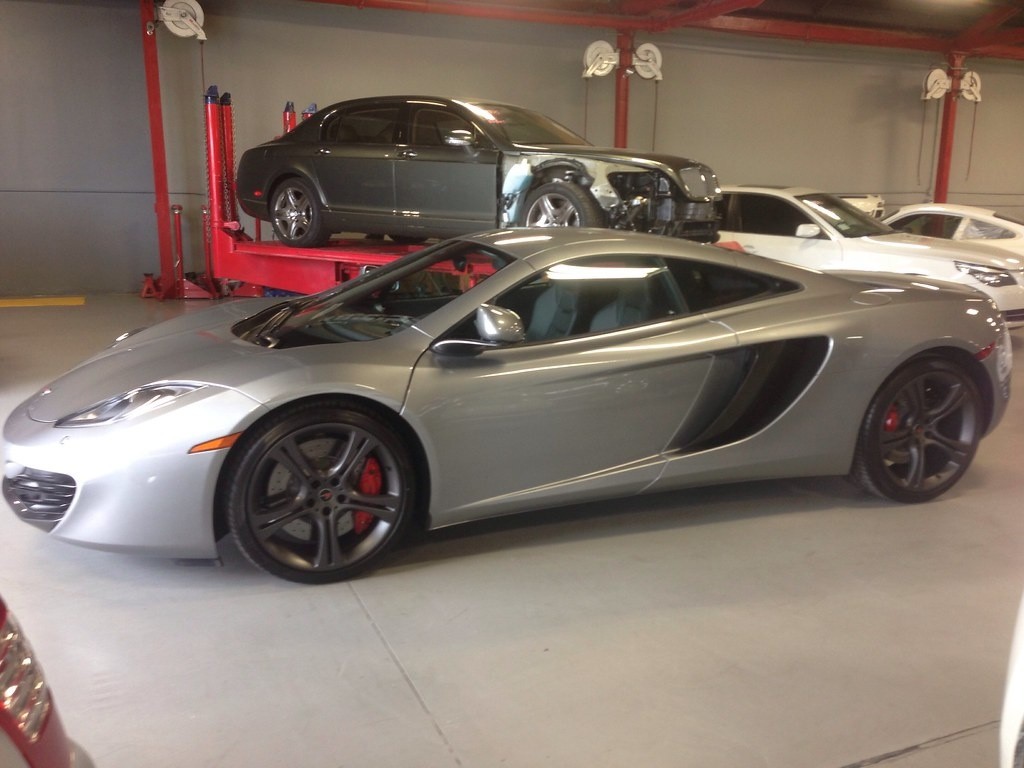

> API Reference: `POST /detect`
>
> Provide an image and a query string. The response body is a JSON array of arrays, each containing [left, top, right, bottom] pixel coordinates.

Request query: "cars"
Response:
[[234, 93, 722, 249], [706, 185, 1024, 331]]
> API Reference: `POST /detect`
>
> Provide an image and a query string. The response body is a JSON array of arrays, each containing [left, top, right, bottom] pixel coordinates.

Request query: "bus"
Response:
[[881, 203, 1024, 256]]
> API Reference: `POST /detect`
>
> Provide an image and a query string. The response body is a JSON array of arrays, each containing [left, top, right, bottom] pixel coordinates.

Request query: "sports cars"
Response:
[[1, 226, 1012, 589]]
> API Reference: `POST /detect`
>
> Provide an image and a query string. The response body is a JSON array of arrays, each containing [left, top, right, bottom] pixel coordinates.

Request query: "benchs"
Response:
[[337, 121, 398, 140]]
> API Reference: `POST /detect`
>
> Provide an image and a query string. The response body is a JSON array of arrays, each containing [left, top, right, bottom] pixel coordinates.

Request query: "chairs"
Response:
[[525, 273, 588, 338], [585, 271, 662, 331]]
